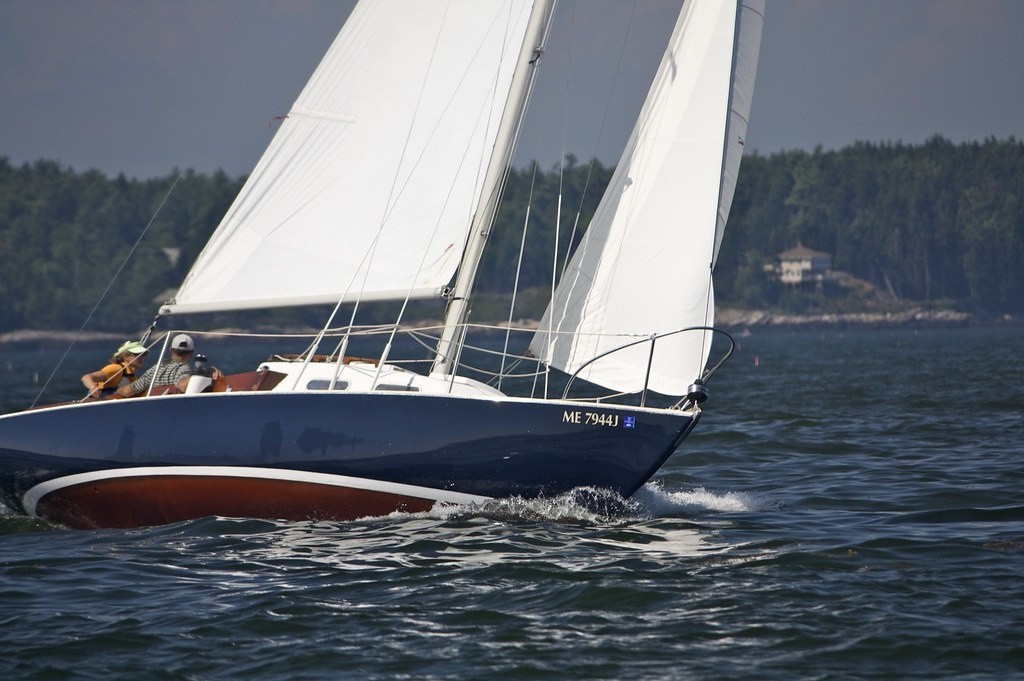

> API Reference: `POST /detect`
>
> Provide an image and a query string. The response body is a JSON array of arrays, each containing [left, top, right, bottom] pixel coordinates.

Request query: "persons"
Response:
[[114, 334, 225, 397], [80, 339, 151, 399]]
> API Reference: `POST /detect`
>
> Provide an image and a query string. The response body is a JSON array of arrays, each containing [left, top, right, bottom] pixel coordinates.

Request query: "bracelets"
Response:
[[91, 384, 97, 389]]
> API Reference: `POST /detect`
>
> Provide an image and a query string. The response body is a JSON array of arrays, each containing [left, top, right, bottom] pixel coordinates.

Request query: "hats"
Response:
[[172, 334, 194, 350], [118, 342, 149, 354]]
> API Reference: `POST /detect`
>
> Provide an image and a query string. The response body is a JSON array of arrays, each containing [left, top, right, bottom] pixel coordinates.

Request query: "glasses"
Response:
[[133, 351, 147, 355]]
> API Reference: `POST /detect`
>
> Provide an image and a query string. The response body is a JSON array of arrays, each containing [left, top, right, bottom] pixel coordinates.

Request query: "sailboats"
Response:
[[0, 0, 774, 535]]
